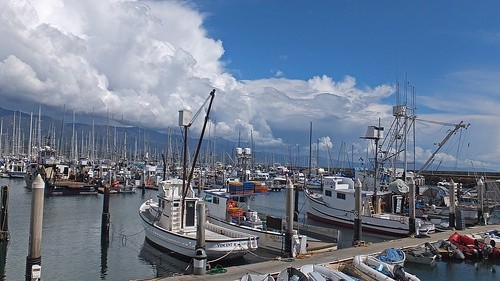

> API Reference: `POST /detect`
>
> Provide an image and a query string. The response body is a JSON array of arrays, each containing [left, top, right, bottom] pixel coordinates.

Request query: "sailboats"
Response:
[[1, 74, 500, 280]]
